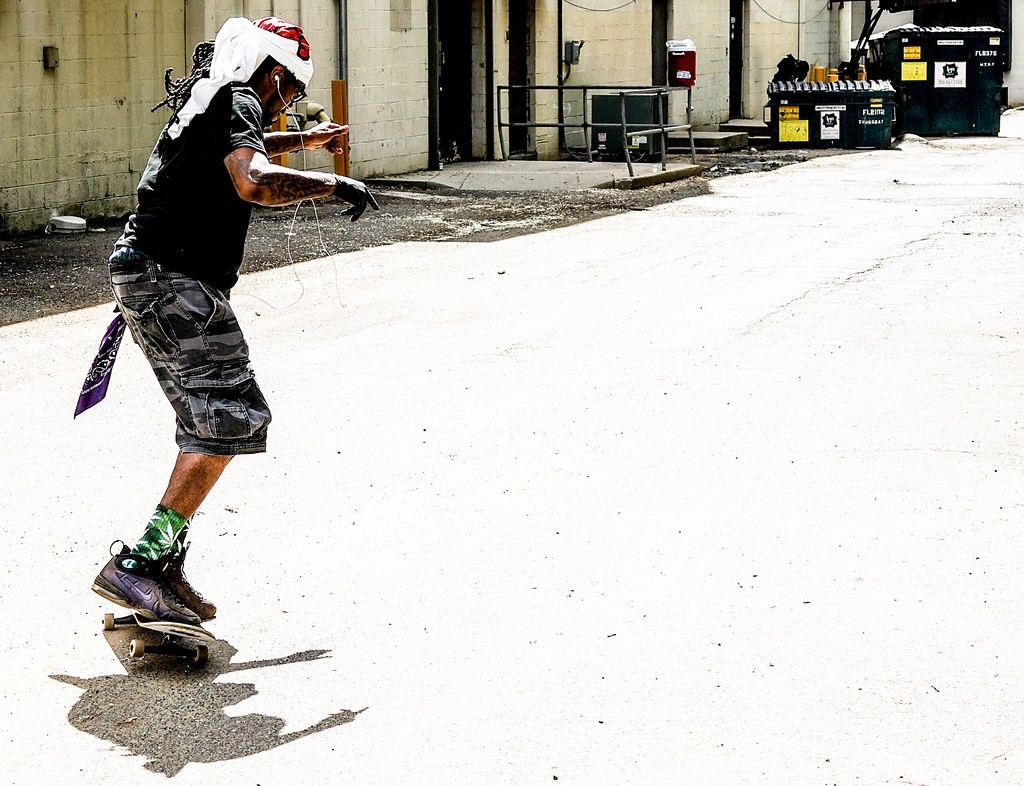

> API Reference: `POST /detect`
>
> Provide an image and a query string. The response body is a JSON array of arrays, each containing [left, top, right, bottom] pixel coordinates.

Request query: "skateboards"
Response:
[[101, 603, 221, 668]]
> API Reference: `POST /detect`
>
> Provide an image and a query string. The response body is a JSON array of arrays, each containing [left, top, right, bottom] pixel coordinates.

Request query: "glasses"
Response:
[[286, 73, 308, 103]]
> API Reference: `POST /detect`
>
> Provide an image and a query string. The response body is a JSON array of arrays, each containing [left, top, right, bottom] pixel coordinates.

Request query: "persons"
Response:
[[91, 18, 380, 625]]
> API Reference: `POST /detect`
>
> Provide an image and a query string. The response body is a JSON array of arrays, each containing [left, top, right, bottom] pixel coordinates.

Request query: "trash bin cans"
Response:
[[762, 78, 897, 150], [881, 25, 1007, 137]]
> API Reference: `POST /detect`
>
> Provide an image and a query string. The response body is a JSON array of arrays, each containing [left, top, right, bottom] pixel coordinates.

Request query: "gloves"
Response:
[[331, 172, 381, 223]]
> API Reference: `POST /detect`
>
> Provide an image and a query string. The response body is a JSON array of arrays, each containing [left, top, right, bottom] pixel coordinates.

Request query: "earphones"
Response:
[[275, 75, 280, 89]]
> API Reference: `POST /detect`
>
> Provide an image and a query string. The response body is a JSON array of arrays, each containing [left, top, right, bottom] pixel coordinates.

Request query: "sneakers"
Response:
[[166, 540, 217, 619], [91, 540, 202, 627]]
